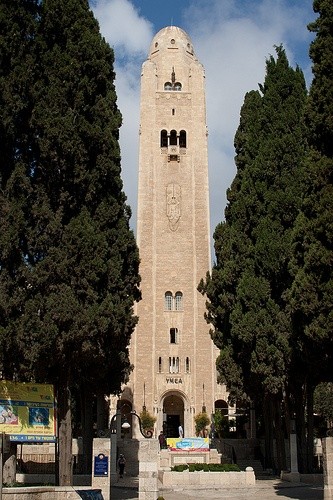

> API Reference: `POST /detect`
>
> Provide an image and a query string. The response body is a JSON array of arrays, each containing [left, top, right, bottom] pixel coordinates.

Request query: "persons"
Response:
[[178, 425, 183, 438], [117, 452, 127, 478]]
